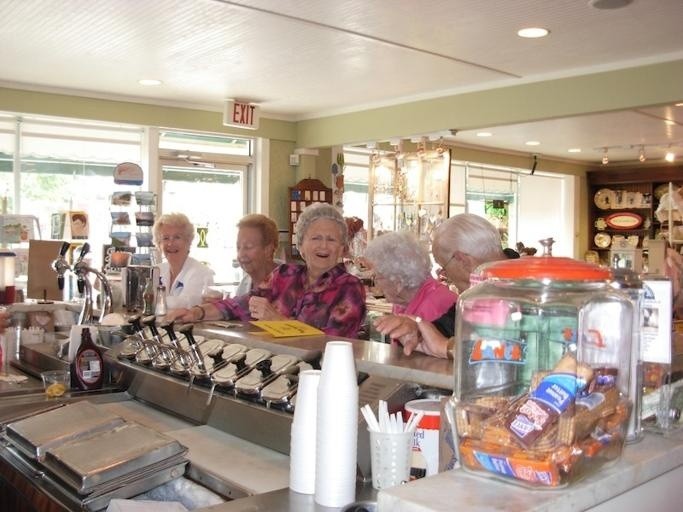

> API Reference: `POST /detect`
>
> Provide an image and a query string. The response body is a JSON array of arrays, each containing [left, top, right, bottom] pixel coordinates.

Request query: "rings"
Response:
[[415, 315, 422, 323]]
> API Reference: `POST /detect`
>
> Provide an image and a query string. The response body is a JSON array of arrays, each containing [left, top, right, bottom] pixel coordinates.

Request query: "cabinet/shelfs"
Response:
[[586, 166, 683, 267]]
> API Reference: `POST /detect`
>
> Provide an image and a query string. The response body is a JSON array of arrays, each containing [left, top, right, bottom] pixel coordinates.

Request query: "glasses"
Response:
[[436, 251, 458, 282]]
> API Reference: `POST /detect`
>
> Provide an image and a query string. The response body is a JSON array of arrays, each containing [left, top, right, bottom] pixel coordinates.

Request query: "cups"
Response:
[[41, 369, 68, 398], [367, 425, 415, 495]]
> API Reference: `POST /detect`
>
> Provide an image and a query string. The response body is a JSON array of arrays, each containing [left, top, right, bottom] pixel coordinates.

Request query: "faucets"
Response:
[[72, 242, 114, 325], [51, 240, 96, 358]]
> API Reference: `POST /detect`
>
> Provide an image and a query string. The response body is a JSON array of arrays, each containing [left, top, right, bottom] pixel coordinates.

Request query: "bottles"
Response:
[[155, 275, 168, 316], [74, 325, 103, 389], [289, 339, 358, 510], [143, 276, 154, 315]]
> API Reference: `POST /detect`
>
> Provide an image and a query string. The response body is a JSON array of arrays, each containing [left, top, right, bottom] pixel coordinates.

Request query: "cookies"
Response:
[[453, 355, 634, 488]]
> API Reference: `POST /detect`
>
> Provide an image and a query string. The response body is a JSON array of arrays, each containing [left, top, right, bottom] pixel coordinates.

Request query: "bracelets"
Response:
[[193, 304, 206, 319], [446, 338, 455, 360]]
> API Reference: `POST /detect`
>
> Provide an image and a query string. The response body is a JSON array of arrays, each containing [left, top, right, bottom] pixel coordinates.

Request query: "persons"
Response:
[[371, 212, 509, 363], [640, 307, 659, 327], [366, 230, 460, 338], [229, 212, 288, 299], [144, 210, 215, 313], [155, 200, 364, 337], [69, 213, 86, 237]]
